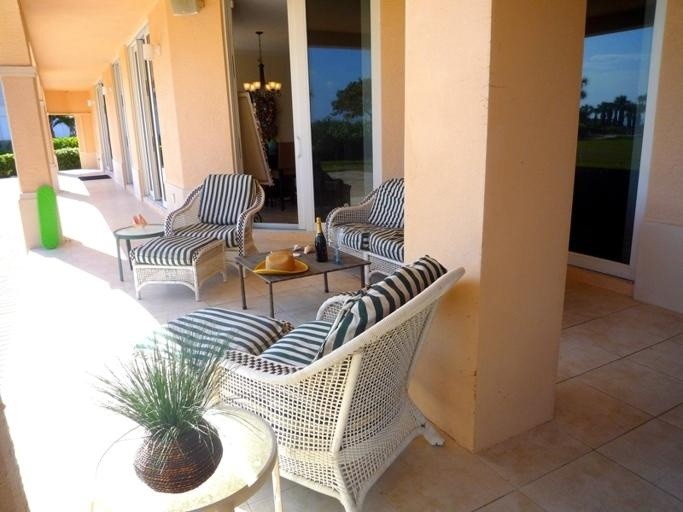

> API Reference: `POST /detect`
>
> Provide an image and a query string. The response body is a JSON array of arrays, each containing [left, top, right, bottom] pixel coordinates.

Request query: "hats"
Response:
[[252, 251, 308, 275]]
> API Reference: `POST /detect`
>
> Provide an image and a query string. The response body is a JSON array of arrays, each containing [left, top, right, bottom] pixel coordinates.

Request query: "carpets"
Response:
[[77, 175, 110, 181]]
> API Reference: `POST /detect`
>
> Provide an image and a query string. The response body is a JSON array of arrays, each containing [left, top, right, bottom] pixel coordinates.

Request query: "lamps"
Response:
[[243, 28, 281, 95], [140, 43, 160, 62], [100, 86, 111, 97]]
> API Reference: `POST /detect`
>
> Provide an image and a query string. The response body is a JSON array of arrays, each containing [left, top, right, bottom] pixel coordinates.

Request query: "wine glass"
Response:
[[328, 226, 346, 266]]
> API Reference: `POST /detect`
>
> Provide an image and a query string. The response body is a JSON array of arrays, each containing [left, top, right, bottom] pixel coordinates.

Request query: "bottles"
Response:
[[314, 217, 328, 263]]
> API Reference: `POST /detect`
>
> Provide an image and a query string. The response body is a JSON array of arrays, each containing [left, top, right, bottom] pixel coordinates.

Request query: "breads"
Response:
[[265, 250, 294, 271]]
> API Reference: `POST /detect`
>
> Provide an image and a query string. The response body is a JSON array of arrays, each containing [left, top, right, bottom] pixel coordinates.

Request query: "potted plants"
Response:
[[90, 322, 242, 492]]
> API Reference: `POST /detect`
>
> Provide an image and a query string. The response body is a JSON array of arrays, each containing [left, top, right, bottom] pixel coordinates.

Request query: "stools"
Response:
[[123, 306, 294, 392], [128, 235, 226, 302]]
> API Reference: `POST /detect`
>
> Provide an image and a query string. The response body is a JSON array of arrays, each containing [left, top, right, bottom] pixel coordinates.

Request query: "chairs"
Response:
[[219, 253, 466, 512], [165, 173, 264, 280]]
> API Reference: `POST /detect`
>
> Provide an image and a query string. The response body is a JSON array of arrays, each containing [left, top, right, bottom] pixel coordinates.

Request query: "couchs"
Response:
[[324, 178, 407, 285]]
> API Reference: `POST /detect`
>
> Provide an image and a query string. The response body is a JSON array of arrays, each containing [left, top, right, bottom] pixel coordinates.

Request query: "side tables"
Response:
[[88, 408, 281, 511], [113, 222, 164, 282]]
